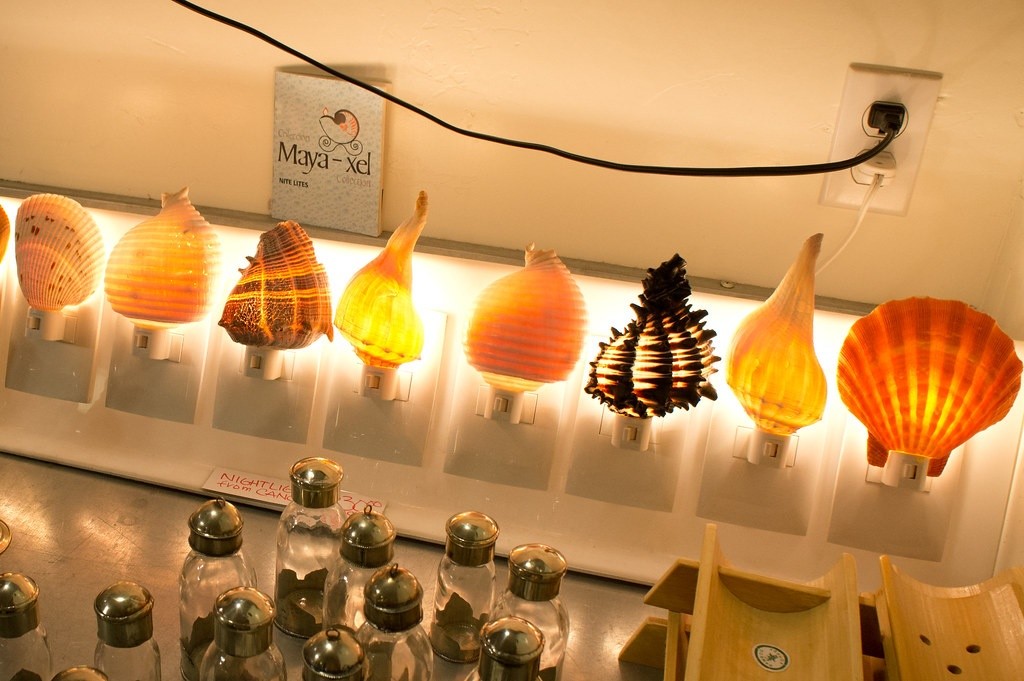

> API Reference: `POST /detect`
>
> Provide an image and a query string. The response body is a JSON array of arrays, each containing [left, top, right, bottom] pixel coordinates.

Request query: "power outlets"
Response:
[[819, 61, 942, 219]]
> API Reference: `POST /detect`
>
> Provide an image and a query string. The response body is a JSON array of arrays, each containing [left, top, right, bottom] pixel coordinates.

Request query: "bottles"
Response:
[[93, 581, 162, 681], [273, 456, 348, 639], [199, 587, 288, 681], [428, 510, 500, 664], [50, 664, 108, 681], [301, 627, 366, 681], [487, 542, 571, 681], [0, 571, 54, 681], [464, 616, 546, 681], [179, 496, 259, 681], [320, 505, 397, 632], [352, 562, 434, 681]]
